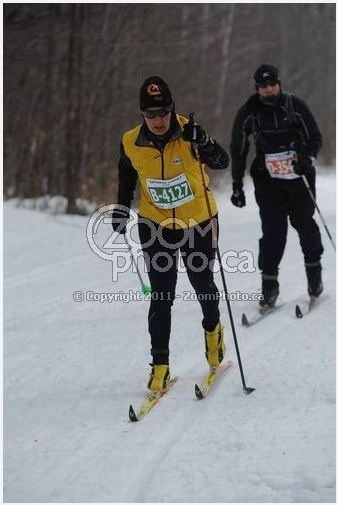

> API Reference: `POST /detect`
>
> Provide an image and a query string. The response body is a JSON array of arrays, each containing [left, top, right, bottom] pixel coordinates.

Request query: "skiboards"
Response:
[[241, 291, 330, 326], [129, 360, 232, 422]]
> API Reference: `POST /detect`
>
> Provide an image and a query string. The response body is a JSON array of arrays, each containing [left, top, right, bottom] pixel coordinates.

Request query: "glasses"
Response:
[[141, 106, 172, 119], [255, 79, 280, 88]]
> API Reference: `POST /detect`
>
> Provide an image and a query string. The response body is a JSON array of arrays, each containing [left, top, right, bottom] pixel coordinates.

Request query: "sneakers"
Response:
[[304, 256, 323, 297], [201, 320, 225, 368], [147, 363, 170, 393], [261, 268, 280, 306]]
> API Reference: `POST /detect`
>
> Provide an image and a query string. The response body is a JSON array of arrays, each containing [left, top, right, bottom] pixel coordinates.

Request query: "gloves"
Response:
[[112, 202, 131, 234], [231, 182, 246, 208], [291, 156, 315, 175], [182, 124, 206, 144]]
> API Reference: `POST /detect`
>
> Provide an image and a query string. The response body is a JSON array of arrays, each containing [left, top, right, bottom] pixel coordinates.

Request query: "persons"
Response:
[[112, 77, 229, 390], [229, 64, 324, 309]]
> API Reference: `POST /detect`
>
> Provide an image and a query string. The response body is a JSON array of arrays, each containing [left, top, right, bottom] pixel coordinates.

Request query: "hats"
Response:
[[254, 63, 278, 81], [139, 76, 172, 111]]
